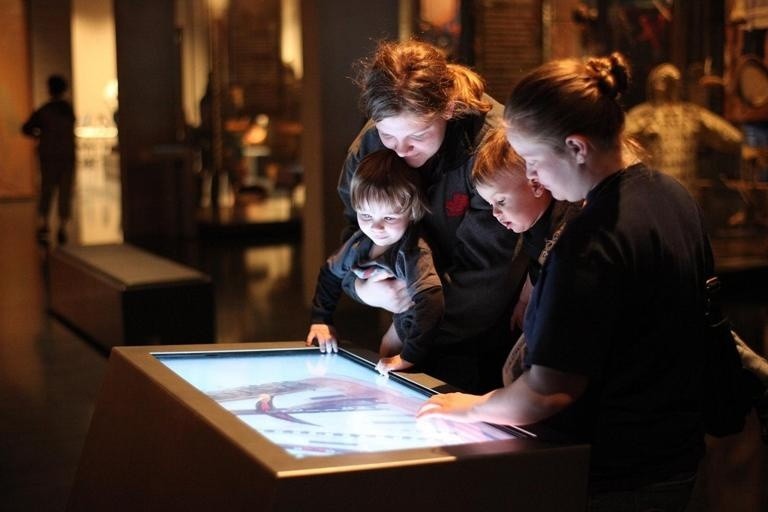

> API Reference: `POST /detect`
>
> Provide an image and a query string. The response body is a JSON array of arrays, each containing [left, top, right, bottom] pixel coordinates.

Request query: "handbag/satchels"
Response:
[[670, 177, 755, 440]]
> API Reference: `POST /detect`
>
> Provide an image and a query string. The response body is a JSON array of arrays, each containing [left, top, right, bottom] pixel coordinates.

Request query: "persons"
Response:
[[468, 124, 579, 332], [19, 72, 77, 252], [198, 71, 263, 211], [616, 61, 744, 205], [304, 146, 445, 381], [730, 330, 768, 379], [424, 52, 715, 512], [334, 38, 514, 392]]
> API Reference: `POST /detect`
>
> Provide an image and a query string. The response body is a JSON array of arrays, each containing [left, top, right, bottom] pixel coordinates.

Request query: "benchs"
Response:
[[47, 242, 218, 355]]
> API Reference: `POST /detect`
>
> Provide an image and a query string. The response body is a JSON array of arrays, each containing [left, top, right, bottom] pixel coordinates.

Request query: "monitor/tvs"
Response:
[[149, 345, 535, 459]]
